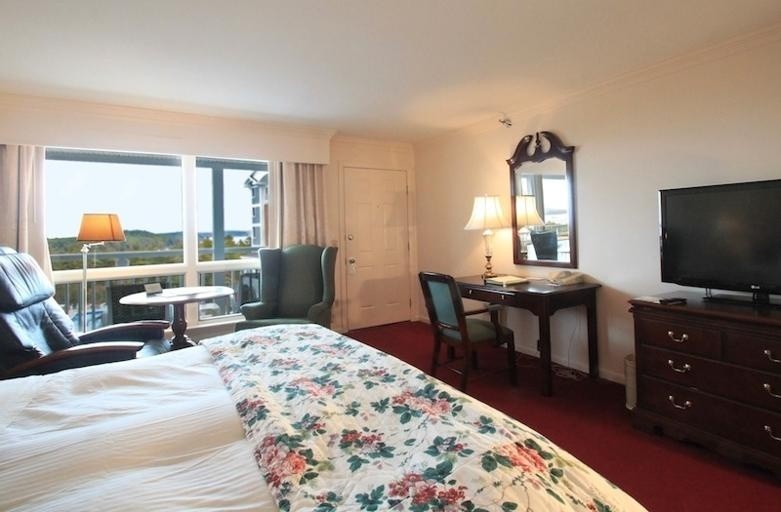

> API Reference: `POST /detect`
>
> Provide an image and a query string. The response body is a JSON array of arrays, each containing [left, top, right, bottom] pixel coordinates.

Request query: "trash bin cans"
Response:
[[623, 353, 637, 411]]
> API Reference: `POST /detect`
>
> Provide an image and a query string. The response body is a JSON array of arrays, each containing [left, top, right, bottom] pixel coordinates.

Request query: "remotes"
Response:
[[659, 297, 687, 304]]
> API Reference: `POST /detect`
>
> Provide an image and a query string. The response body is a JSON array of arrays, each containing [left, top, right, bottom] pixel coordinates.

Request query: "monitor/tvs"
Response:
[[657, 179, 781, 309]]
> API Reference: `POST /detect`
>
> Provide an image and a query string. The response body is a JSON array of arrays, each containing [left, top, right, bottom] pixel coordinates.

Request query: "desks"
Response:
[[453, 275, 604, 396], [119, 286, 237, 351]]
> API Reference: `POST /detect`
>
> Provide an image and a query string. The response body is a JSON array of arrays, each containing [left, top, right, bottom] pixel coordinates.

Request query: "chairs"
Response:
[[416, 271, 516, 399], [236, 245, 339, 331], [97, 280, 166, 329], [530, 231, 558, 262], [0, 246, 176, 379]]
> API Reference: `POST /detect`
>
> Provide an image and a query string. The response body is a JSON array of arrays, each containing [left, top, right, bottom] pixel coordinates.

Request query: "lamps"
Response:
[[517, 197, 544, 262], [464, 195, 508, 279], [77, 213, 126, 332]]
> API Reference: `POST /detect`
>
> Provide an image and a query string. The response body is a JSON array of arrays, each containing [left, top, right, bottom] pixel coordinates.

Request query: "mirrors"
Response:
[[507, 132, 578, 268]]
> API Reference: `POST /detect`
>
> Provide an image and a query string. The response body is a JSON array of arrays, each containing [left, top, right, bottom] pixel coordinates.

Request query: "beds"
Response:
[[0, 324, 651, 511]]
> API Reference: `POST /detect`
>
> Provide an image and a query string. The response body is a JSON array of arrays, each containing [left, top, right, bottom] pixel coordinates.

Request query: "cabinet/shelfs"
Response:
[[627, 291, 781, 477]]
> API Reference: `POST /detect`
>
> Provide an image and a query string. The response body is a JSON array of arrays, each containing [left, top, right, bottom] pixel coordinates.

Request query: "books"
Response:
[[630, 294, 686, 305], [484, 275, 527, 286]]
